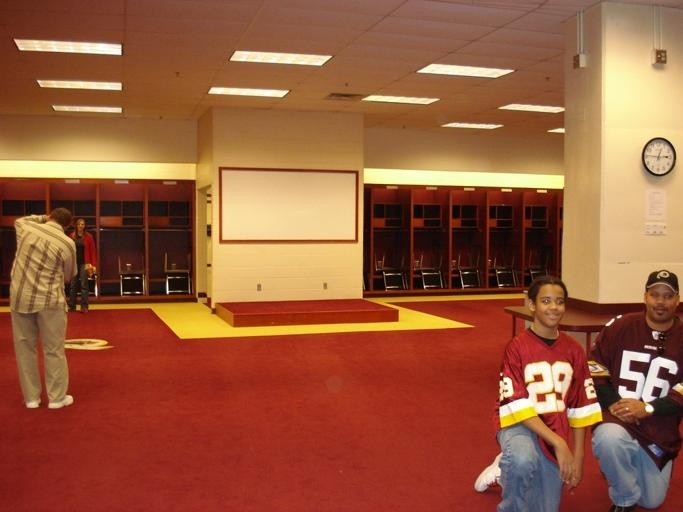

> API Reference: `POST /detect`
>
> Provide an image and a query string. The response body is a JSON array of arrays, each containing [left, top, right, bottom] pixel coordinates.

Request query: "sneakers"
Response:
[[48, 394, 74, 409], [67, 306, 88, 313], [474, 451, 504, 493], [608, 503, 637, 512], [25, 396, 42, 408]]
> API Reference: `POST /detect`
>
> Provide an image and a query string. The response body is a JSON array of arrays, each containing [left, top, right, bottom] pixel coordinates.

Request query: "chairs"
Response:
[[76, 252, 192, 297], [373, 250, 553, 291]]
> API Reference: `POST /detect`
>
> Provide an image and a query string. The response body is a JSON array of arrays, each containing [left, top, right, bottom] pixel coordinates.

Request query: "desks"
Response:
[[504, 302, 617, 358]]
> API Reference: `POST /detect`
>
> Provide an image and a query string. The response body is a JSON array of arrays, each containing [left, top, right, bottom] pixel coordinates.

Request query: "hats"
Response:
[[645, 269, 679, 294]]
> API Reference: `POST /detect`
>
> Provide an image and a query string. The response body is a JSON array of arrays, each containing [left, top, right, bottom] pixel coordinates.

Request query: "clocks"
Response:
[[641, 137, 677, 176]]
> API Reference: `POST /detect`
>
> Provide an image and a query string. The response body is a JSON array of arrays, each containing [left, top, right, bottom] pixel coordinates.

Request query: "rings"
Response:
[[625, 407, 629, 411]]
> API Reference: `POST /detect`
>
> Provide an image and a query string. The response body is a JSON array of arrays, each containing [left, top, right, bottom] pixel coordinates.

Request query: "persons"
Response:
[[65, 218, 95, 313], [473, 275, 603, 512], [587, 269, 682, 511], [9, 207, 79, 409]]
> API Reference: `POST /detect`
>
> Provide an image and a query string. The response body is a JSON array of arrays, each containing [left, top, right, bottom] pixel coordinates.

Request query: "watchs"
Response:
[[644, 402, 654, 414]]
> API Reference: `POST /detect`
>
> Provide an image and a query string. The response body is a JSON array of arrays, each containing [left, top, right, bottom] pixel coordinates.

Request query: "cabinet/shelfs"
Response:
[[370, 202, 564, 232], [0, 198, 193, 233]]
